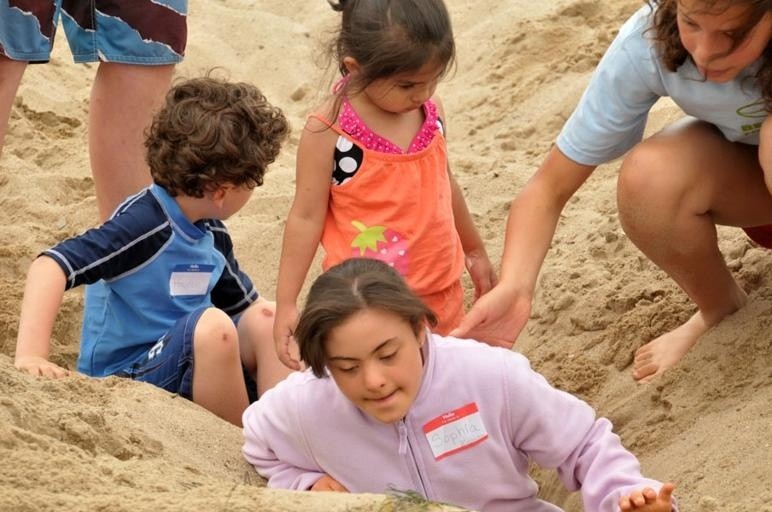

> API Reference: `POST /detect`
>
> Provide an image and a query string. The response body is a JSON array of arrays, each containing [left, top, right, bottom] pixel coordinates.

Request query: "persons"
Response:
[[242, 259, 675, 512], [273, 0, 500, 371], [448, 0, 772, 386], [14, 66, 308, 429], [0, 1, 188, 226]]
